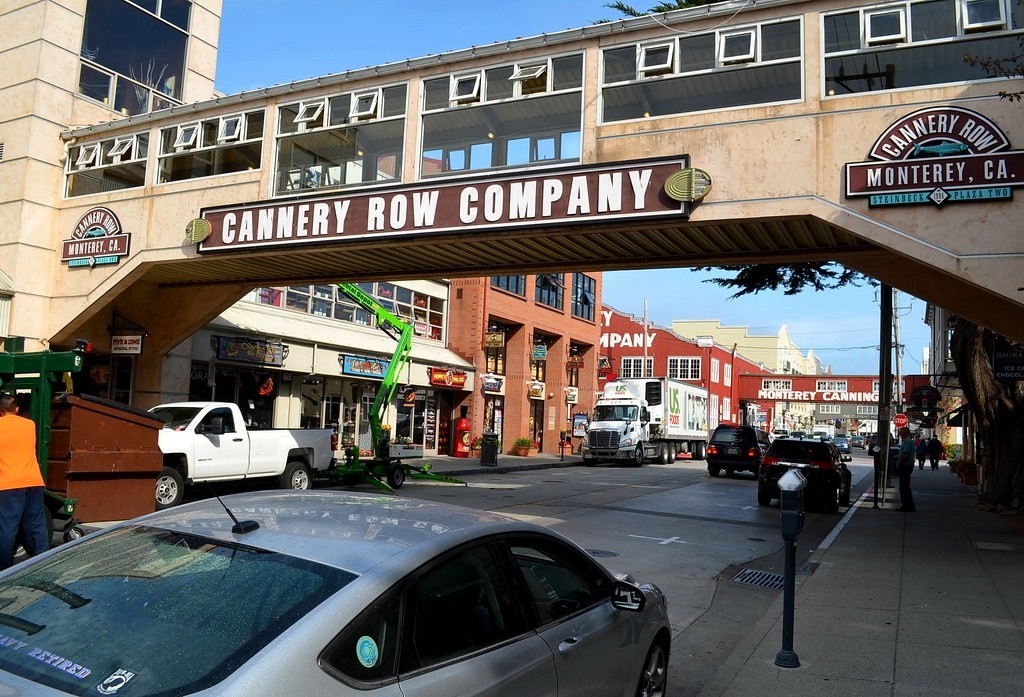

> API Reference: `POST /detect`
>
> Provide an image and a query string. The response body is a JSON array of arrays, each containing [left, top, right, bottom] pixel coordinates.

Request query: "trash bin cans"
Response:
[[480, 432, 500, 466], [889, 447, 901, 476]]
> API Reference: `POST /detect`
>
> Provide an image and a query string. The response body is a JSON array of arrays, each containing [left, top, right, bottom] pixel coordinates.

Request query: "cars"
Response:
[[834, 433, 846, 438], [806, 434, 813, 440], [868, 433, 895, 456], [851, 436, 865, 447], [790, 431, 806, 439], [832, 437, 851, 454], [812, 431, 826, 437], [773, 429, 789, 439], [827, 436, 834, 443], [0, 490, 671, 697]]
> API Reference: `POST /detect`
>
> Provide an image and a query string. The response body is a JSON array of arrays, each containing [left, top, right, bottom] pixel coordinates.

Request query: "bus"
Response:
[[330, 284, 469, 495]]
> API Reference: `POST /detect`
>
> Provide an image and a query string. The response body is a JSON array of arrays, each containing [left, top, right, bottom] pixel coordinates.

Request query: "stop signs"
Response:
[[893, 414, 908, 428]]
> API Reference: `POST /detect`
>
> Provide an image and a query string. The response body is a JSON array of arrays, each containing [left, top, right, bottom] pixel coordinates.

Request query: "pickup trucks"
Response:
[[147, 402, 333, 510]]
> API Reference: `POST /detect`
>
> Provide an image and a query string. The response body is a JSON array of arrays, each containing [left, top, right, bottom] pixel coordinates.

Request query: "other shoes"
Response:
[[896, 506, 918, 512], [919, 466, 924, 471], [935, 463, 939, 470], [932, 467, 936, 471]]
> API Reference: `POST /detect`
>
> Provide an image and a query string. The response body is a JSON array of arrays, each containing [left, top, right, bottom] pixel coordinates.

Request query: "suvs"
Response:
[[758, 431, 852, 516], [707, 424, 770, 479]]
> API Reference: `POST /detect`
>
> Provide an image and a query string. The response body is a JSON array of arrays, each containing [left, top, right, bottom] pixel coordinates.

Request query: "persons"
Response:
[[896, 427, 916, 511], [915, 439, 927, 470], [0, 396, 49, 569], [927, 435, 943, 469], [914, 437, 920, 447]]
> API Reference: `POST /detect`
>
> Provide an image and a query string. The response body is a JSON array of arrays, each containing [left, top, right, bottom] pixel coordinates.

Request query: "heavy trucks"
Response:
[[582, 376, 710, 468]]
